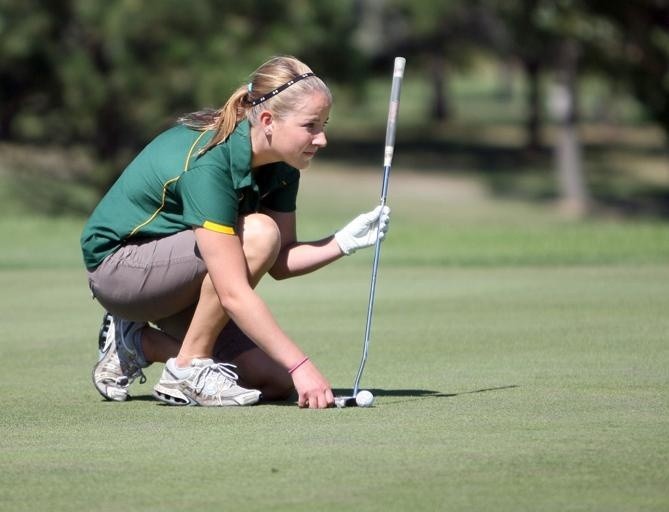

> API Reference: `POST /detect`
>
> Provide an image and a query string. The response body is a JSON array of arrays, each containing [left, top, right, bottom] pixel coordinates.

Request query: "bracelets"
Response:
[[287, 355, 309, 377]]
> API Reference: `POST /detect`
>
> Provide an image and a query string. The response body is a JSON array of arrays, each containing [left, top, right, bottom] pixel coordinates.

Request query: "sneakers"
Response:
[[153, 357, 263, 407], [92, 312, 147, 401]]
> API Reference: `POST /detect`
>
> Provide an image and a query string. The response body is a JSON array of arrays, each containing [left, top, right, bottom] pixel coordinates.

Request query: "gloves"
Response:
[[334, 206, 390, 256]]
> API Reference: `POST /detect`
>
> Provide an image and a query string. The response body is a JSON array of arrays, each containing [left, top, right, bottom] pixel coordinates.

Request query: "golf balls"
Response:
[[356, 391, 375, 407]]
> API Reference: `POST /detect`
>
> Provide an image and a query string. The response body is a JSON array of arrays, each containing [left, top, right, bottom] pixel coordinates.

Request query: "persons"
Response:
[[80, 54, 396, 411]]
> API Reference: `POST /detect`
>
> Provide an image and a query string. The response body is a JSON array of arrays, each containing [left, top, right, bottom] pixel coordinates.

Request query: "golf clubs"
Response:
[[332, 57, 406, 408]]
